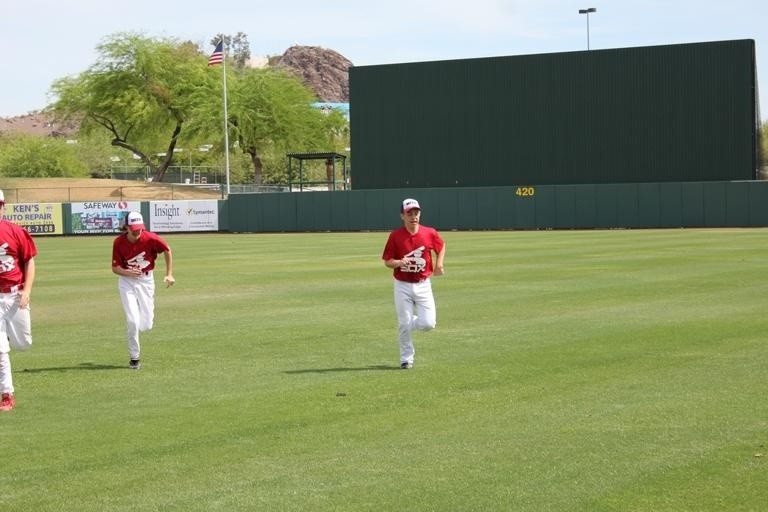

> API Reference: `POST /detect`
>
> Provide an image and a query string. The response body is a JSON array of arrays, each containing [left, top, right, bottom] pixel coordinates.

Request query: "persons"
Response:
[[0, 190, 37, 412], [382, 199, 445, 368], [112, 212, 175, 369]]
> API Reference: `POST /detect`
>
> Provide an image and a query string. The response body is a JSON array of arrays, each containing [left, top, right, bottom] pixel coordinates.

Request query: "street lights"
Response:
[[579, 8, 597, 50]]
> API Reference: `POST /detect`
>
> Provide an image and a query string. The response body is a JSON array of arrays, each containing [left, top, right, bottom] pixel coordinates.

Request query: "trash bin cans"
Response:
[[148, 178, 152, 182], [185, 179, 190, 184]]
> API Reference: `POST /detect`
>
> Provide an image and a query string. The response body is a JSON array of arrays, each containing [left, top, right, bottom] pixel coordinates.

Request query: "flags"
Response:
[[208, 41, 223, 66]]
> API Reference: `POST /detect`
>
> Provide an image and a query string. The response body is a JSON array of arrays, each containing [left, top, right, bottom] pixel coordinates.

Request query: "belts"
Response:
[[393, 276, 429, 284], [1, 284, 22, 293]]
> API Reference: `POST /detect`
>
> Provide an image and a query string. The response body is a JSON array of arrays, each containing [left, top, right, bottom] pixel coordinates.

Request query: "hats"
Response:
[[126, 211, 146, 231], [402, 199, 422, 212], [0, 190, 5, 202]]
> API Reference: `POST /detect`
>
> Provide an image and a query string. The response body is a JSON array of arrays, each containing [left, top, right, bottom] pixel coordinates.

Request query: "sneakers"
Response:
[[400, 363, 413, 369], [130, 357, 139, 368], [1, 394, 15, 410]]
